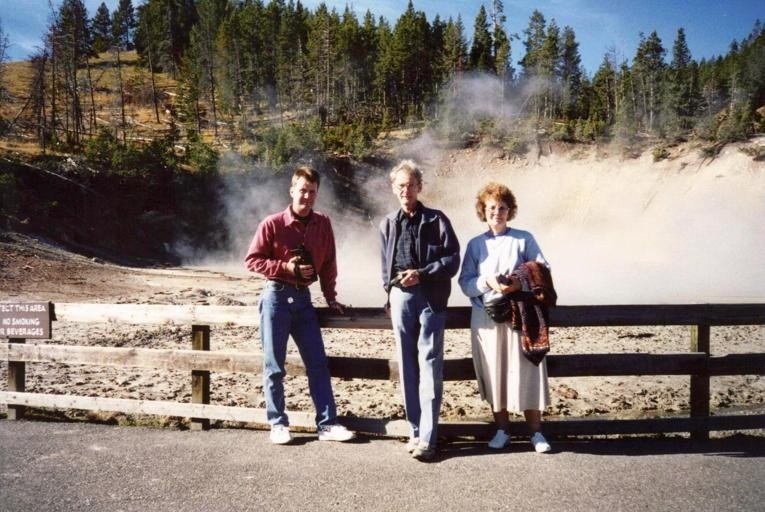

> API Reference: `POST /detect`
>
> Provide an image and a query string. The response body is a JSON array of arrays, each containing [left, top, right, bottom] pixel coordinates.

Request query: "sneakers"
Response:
[[403, 437, 437, 464], [269, 422, 292, 445], [529, 432, 550, 454], [489, 429, 509, 451], [316, 420, 353, 443]]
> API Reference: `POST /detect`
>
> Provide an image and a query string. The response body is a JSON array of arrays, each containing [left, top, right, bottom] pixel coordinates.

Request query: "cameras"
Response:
[[496, 273, 514, 287], [289, 245, 319, 284], [389, 270, 403, 288]]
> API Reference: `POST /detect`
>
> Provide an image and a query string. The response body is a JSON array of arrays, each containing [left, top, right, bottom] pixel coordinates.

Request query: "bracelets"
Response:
[[329, 300, 336, 306]]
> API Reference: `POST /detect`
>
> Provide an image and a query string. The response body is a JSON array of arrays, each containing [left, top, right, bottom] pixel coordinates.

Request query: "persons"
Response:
[[377, 160, 461, 461], [458, 180, 554, 454], [242, 164, 358, 446]]
